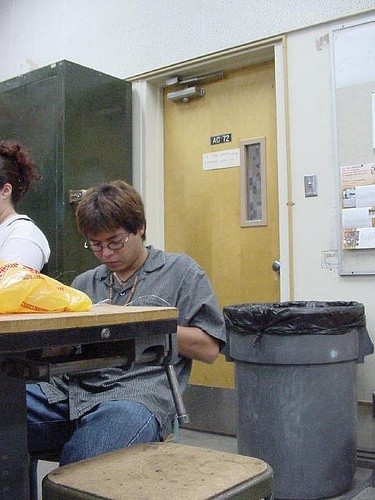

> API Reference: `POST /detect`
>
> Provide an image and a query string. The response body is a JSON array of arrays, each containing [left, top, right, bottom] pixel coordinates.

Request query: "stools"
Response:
[[41, 442, 275, 500]]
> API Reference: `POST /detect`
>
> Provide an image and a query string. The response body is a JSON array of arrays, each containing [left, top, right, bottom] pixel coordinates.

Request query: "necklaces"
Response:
[[109, 272, 139, 305]]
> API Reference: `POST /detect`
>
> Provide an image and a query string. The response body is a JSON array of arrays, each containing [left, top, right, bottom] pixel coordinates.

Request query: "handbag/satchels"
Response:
[[0, 257, 92, 314]]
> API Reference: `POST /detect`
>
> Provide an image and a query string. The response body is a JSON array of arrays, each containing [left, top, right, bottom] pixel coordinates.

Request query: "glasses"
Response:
[[82, 231, 132, 252]]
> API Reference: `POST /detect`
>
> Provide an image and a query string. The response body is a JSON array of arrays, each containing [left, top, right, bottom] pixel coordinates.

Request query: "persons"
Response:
[[26, 179, 227, 466], [0, 139, 51, 272]]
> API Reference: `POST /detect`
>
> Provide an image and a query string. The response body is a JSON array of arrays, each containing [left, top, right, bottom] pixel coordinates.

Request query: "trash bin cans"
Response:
[[222, 300, 373, 500]]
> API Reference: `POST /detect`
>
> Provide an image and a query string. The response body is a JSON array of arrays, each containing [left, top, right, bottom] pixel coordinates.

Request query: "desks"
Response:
[[0, 305, 179, 500]]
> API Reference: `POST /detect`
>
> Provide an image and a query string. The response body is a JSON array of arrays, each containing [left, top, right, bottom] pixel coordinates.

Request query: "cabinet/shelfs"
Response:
[[1, 59, 133, 287]]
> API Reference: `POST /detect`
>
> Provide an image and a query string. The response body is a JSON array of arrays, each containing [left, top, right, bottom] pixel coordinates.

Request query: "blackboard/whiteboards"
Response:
[[328, 18, 374, 276]]
